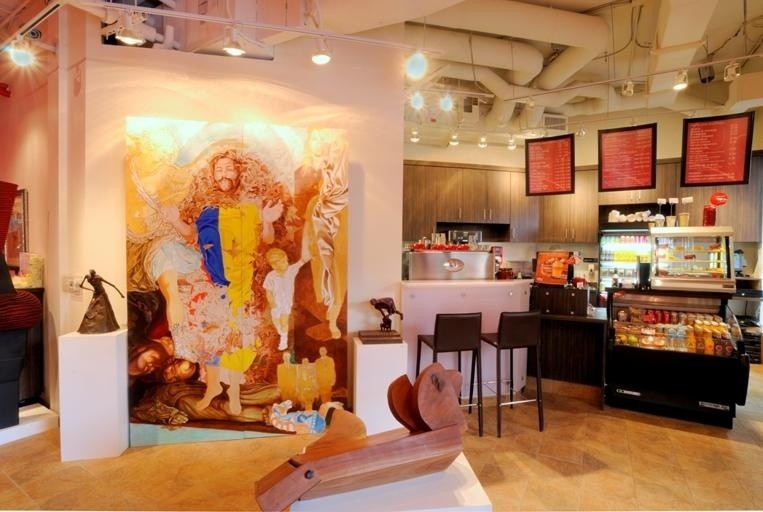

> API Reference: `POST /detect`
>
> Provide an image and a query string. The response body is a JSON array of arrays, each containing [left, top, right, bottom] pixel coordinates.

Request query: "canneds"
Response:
[[645, 310, 724, 323], [670, 324, 733, 358]]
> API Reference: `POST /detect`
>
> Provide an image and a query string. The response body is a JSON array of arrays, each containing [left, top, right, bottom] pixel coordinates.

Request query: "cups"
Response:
[[657, 218, 664, 227], [679, 213, 690, 226], [666, 215, 676, 227]]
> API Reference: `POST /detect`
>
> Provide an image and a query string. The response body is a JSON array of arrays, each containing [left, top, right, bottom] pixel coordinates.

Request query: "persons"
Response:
[[314, 345, 336, 404], [296, 357, 317, 412], [76, 269, 125, 335], [358, 297, 404, 344], [128, 382, 282, 427], [127, 121, 243, 366], [143, 150, 292, 416], [142, 359, 196, 388], [277, 351, 297, 408], [262, 247, 312, 351], [128, 336, 174, 390], [306, 129, 349, 340]]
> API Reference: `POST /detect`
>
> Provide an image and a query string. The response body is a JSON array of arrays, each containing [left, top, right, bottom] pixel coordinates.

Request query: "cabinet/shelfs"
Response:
[[595, 228, 737, 295], [530, 285, 588, 317], [527, 314, 607, 389], [727, 299, 760, 318], [602, 288, 750, 430]]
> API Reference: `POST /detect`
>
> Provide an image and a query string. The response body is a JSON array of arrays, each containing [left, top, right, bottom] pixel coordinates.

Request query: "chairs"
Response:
[[468, 312, 544, 438], [415, 312, 484, 437]]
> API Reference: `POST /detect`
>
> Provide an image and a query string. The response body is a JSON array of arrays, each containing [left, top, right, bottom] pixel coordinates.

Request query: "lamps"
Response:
[[311, 44, 331, 66], [9, 40, 35, 65], [222, 39, 246, 58], [673, 71, 688, 91], [621, 81, 634, 96], [722, 62, 741, 82], [114, 26, 144, 48], [406, 16, 429, 80]]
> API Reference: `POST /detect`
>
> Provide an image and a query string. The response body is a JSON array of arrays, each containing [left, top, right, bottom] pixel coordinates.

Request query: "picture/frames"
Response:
[[679, 110, 755, 187], [525, 133, 575, 197], [2, 188, 29, 270], [596, 122, 657, 192]]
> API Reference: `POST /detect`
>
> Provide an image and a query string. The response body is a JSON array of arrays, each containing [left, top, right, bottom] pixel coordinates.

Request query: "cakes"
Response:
[[655, 243, 727, 278], [614, 322, 669, 349]]
